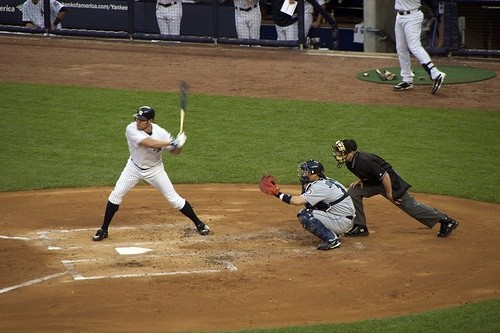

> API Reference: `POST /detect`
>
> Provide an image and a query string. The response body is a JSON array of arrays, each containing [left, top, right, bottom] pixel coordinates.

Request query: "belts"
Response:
[[347, 214, 354, 220], [158, 2, 176, 7], [236, 4, 256, 11], [398, 7, 421, 15], [131, 159, 150, 170]]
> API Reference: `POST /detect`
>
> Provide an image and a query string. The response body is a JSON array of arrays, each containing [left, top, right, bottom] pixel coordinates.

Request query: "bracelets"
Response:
[[281, 194, 291, 204], [53, 17, 61, 26]]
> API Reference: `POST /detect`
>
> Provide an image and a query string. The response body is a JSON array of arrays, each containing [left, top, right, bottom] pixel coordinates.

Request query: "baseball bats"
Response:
[[180, 81, 187, 136], [376, 69, 387, 80], [384, 69, 398, 80]]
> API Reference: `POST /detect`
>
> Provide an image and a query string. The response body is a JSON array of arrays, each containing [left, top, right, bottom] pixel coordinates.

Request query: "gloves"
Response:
[[171, 132, 187, 148]]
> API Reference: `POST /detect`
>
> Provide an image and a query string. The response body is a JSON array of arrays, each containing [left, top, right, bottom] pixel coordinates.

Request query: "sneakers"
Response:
[[432, 72, 446, 93], [198, 222, 209, 235], [317, 237, 341, 250], [437, 217, 459, 237], [344, 224, 368, 236], [394, 81, 413, 90], [93, 228, 108, 240]]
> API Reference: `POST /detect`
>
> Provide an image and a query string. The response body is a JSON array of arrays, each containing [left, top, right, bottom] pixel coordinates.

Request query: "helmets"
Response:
[[133, 105, 155, 120], [297, 159, 324, 184], [332, 140, 356, 168]]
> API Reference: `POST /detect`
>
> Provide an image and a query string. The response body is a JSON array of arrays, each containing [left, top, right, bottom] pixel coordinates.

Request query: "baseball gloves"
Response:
[[260, 175, 279, 196]]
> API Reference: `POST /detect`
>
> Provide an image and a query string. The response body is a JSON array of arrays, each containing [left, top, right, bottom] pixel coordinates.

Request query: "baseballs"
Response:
[[364, 73, 368, 76]]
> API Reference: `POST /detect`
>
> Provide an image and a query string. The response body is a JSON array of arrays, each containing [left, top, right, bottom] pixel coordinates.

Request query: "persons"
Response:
[[274, 0, 299, 42], [22, 0, 65, 29], [394, 0, 446, 95], [155, 0, 183, 35], [260, 159, 356, 251], [92, 105, 209, 240], [234, 0, 262, 47], [333, 138, 459, 238], [303, 0, 326, 39]]
